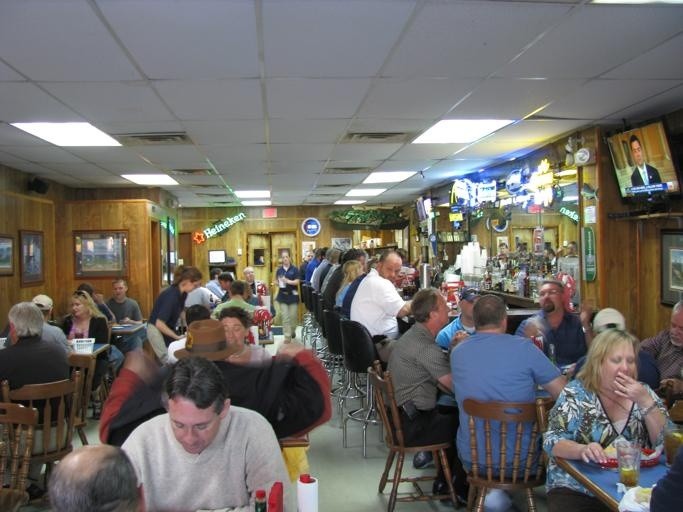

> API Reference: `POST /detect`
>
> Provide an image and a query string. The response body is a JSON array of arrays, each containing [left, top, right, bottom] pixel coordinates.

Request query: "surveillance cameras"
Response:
[[419, 171, 425, 179]]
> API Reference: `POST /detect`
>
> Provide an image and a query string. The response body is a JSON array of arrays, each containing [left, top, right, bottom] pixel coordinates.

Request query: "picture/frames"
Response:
[[168, 216, 178, 286], [160, 218, 168, 287], [73, 228, 131, 280], [0, 235, 15, 277], [660, 227, 683, 307], [19, 229, 44, 288]]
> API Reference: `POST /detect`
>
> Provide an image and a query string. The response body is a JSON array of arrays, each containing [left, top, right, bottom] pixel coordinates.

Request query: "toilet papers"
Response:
[[297, 478, 319, 511]]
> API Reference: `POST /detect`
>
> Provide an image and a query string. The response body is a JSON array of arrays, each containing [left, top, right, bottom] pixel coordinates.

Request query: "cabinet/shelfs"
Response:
[[326, 206, 410, 234]]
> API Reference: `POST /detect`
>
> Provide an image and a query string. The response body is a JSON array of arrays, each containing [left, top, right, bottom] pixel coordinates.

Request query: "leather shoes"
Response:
[[433, 479, 443, 496], [440, 489, 469, 505]]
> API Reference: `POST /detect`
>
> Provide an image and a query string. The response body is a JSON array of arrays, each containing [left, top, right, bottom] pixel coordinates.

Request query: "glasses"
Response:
[[74, 291, 87, 299], [93, 400, 102, 420]]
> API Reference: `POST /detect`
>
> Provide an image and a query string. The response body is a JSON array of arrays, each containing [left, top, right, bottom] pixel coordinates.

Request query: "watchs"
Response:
[[641, 400, 658, 416]]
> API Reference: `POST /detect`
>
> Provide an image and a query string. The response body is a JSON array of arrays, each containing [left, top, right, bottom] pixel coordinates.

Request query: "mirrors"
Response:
[[417, 133, 581, 313]]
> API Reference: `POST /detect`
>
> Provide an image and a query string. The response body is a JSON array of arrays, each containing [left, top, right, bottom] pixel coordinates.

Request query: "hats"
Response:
[[458, 286, 482, 302], [174, 319, 240, 361], [32, 294, 54, 312], [592, 307, 625, 333]]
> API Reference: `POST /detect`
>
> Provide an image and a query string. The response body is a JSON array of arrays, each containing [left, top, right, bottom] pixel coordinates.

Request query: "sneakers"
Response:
[[285, 336, 291, 344], [413, 451, 434, 468], [292, 334, 295, 338]]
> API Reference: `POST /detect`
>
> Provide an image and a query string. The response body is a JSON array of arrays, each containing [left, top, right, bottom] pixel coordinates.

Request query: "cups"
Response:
[[617, 441, 641, 487], [664, 427, 683, 467]]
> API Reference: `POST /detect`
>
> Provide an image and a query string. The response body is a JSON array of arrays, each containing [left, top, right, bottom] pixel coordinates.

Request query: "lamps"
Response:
[[410, 119, 517, 146], [120, 173, 181, 186], [361, 170, 421, 187], [342, 187, 386, 200], [334, 197, 365, 207], [7, 120, 122, 149]]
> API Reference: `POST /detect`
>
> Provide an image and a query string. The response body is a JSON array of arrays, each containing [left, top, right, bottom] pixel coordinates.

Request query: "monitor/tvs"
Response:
[[605, 122, 681, 218], [207, 249, 226, 264]]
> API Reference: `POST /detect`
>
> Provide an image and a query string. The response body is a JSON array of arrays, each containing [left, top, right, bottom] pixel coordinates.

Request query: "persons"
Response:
[[383, 288, 477, 506], [450, 295, 568, 477], [106, 280, 141, 352], [350, 251, 413, 362], [121, 356, 289, 512], [514, 281, 586, 366], [206, 268, 227, 299], [78, 284, 124, 381], [300, 248, 376, 319], [168, 304, 210, 364], [210, 273, 234, 306], [176, 287, 221, 334], [435, 288, 480, 349], [543, 330, 677, 512], [570, 308, 626, 380], [4, 295, 67, 347], [630, 135, 665, 195], [107, 319, 325, 448], [276, 250, 301, 344], [0, 303, 70, 501], [638, 301, 683, 408], [220, 308, 272, 362], [241, 281, 259, 306], [212, 280, 256, 321], [551, 242, 578, 266], [146, 265, 202, 365], [497, 244, 509, 258], [47, 445, 146, 512], [243, 268, 267, 295], [58, 291, 110, 420]]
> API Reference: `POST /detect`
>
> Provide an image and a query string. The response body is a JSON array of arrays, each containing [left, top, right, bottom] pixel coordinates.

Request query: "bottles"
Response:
[[548, 342, 557, 363], [253, 489, 267, 512]]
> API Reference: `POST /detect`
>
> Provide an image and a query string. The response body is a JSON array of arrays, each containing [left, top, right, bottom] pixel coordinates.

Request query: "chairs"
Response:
[[309, 293, 343, 367], [91, 321, 116, 417], [1, 370, 83, 512], [367, 366, 459, 512], [316, 304, 358, 388], [0, 403, 39, 511], [654, 380, 673, 406], [321, 308, 370, 398], [463, 397, 555, 511], [66, 353, 94, 446], [337, 317, 390, 452], [300, 284, 327, 347], [535, 396, 575, 480]]
